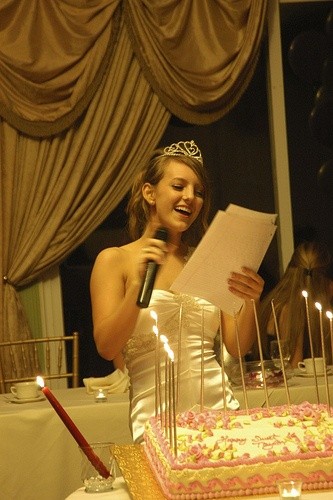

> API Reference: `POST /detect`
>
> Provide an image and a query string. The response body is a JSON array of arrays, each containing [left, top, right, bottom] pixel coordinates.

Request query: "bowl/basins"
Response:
[[222, 359, 289, 385]]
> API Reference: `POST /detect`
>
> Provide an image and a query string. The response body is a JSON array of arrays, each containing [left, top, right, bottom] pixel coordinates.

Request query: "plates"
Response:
[[294, 368, 333, 377], [10, 395, 46, 403]]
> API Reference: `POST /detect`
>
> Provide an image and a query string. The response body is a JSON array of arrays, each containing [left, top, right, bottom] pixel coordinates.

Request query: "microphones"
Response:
[[136, 226, 169, 307]]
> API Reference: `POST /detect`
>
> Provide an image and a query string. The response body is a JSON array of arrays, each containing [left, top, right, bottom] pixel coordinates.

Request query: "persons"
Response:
[[252, 242, 333, 368], [89, 148, 262, 444]]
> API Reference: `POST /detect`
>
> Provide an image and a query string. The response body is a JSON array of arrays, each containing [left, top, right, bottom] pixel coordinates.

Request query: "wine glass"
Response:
[[269, 340, 291, 380]]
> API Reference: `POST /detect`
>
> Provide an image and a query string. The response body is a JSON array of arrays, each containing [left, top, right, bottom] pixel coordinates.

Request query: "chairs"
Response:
[[0, 332, 79, 394]]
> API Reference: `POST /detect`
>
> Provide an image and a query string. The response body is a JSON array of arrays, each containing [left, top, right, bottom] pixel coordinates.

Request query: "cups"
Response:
[[298, 357, 324, 373], [78, 442, 116, 493], [94, 388, 108, 404], [10, 382, 39, 399]]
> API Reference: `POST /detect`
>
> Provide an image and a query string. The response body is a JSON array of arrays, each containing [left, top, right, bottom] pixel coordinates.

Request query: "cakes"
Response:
[[143, 402, 333, 500]]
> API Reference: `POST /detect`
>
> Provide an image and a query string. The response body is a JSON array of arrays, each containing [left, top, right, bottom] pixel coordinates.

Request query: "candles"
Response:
[[36, 375, 111, 478], [155, 299, 333, 458]]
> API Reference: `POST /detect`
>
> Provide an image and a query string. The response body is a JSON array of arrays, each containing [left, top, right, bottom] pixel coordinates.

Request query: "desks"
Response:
[[0, 365, 333, 500]]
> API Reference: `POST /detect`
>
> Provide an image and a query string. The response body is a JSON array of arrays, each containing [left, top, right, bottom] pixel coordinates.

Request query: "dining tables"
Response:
[[65, 476, 333, 500]]
